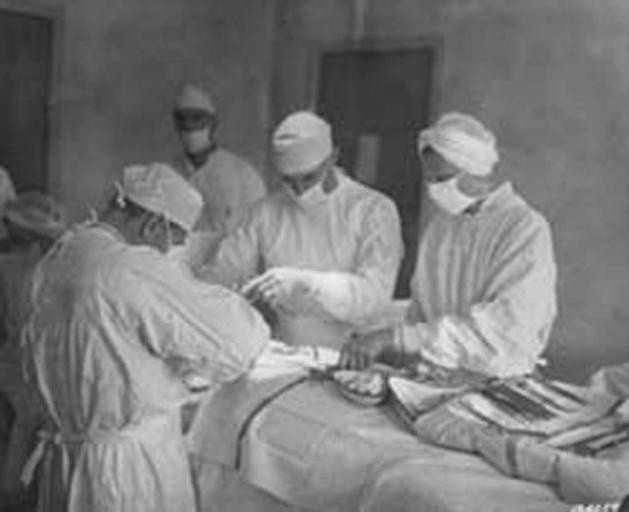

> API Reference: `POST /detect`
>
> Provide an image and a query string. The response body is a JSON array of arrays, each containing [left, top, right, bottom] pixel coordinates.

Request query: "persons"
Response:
[[144, 81, 267, 282], [337, 110, 560, 388], [18, 160, 271, 512], [0, 191, 76, 512], [197, 111, 406, 352]]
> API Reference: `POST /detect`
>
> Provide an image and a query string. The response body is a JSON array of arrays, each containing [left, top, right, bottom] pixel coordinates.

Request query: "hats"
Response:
[[120, 158, 205, 236], [416, 108, 499, 178], [267, 109, 334, 178], [171, 80, 220, 118]]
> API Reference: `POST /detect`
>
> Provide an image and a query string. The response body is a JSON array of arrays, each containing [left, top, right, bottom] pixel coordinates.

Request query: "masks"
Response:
[[154, 212, 192, 262], [177, 124, 217, 157], [420, 169, 485, 217], [277, 167, 345, 212]]
